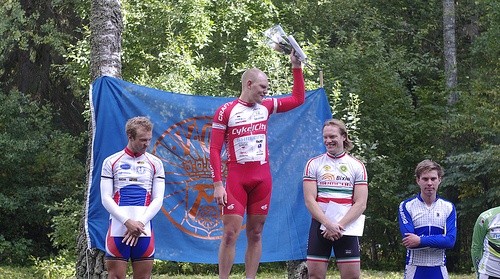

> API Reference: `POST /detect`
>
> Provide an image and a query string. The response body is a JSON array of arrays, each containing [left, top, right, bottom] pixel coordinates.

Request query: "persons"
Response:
[[398, 159, 458, 279], [470, 205, 500, 279], [209, 48, 306, 279], [302, 118, 369, 279], [99, 116, 166, 279]]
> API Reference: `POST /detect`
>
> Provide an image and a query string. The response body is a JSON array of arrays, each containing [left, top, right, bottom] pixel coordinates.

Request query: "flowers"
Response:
[[263, 25, 320, 77]]
